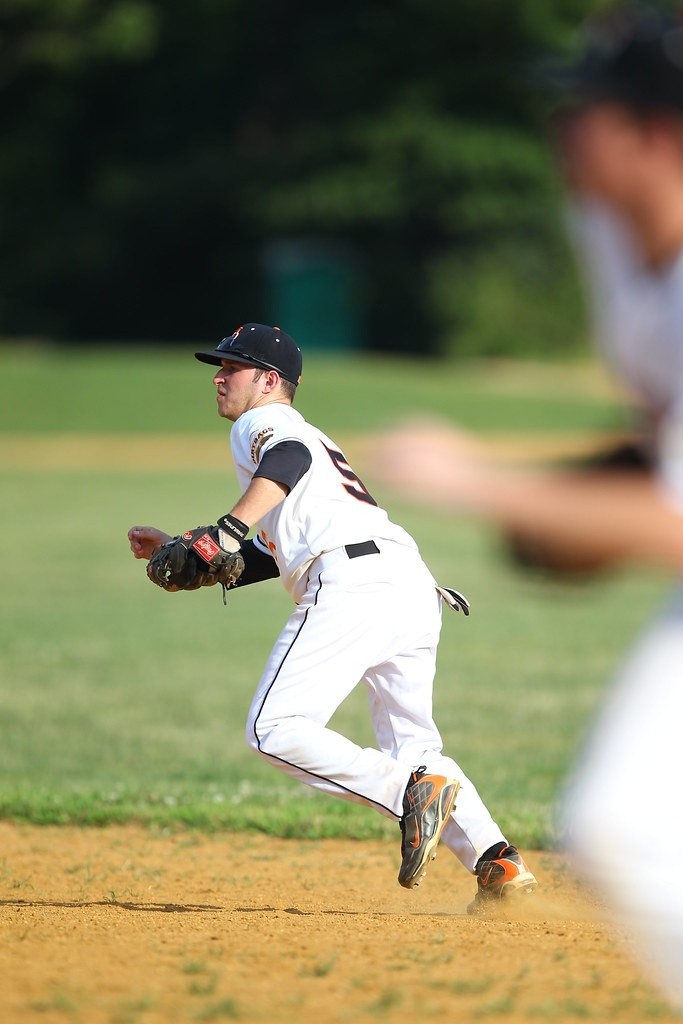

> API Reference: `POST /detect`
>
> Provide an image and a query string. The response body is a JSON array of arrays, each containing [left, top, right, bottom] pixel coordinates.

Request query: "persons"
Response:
[[128, 323, 541, 916], [384, 37, 678, 1016]]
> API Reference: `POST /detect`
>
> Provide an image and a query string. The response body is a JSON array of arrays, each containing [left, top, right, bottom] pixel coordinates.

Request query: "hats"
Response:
[[193, 321, 304, 390]]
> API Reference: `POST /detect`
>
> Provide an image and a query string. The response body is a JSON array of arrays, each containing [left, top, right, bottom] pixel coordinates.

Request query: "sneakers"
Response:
[[396, 762, 460, 889], [463, 844, 538, 916]]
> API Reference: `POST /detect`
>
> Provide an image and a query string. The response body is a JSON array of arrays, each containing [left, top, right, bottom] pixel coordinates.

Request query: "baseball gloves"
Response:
[[145, 522, 245, 605]]
